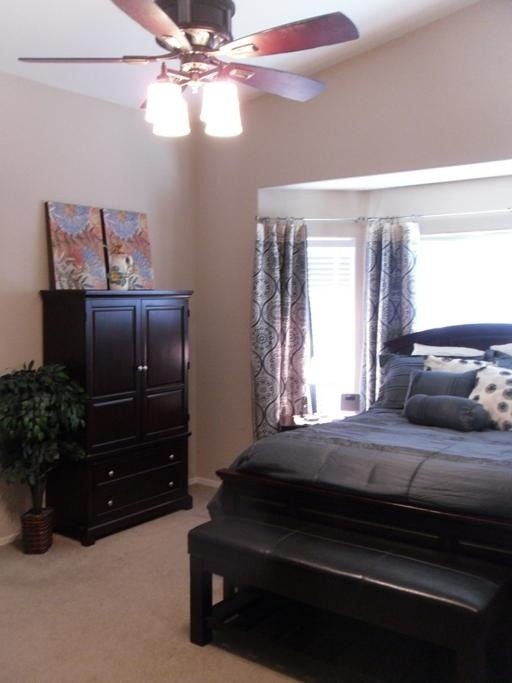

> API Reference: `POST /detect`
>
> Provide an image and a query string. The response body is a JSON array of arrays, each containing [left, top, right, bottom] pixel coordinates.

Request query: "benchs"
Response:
[[188, 509, 512, 683]]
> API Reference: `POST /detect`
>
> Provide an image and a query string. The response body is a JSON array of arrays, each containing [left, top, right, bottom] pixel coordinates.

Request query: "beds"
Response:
[[218, 323, 511, 516]]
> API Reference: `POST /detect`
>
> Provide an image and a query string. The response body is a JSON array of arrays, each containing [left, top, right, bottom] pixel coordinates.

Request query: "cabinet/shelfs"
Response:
[[38, 288, 196, 547]]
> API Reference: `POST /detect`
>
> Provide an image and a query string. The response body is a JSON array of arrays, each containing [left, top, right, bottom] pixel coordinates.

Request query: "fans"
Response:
[[16, 1, 360, 111]]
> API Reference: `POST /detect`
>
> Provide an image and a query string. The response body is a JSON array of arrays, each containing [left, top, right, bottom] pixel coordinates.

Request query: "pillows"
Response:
[[377, 340, 511, 433]]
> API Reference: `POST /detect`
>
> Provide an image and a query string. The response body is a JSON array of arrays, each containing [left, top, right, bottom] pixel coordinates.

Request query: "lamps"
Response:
[[144, 76, 245, 141]]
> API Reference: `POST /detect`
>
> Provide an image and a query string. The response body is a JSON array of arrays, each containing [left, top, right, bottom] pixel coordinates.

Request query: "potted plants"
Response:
[[1, 358, 88, 553]]
